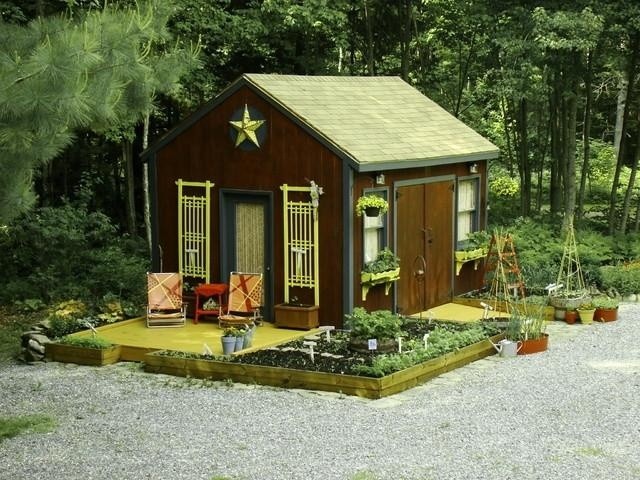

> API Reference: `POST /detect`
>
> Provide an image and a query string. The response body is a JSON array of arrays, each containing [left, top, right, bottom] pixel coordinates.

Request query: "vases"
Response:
[[273, 302, 319, 330]]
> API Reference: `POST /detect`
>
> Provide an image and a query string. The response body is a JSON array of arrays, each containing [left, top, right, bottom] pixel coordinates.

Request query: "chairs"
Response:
[[217, 270, 264, 329], [145, 272, 187, 328]]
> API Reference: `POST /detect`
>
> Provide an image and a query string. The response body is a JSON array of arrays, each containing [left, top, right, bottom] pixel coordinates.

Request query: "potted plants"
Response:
[[553, 291, 621, 325], [50, 334, 123, 366], [220, 324, 254, 354], [503, 303, 549, 355], [355, 194, 390, 218], [454, 230, 493, 277], [360, 245, 401, 302]]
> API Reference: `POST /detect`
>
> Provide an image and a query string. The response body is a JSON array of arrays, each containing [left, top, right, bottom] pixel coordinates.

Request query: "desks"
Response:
[[193, 283, 228, 326]]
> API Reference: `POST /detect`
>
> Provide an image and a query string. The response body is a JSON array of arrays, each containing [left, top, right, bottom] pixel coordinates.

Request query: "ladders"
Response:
[[478, 233, 527, 314]]
